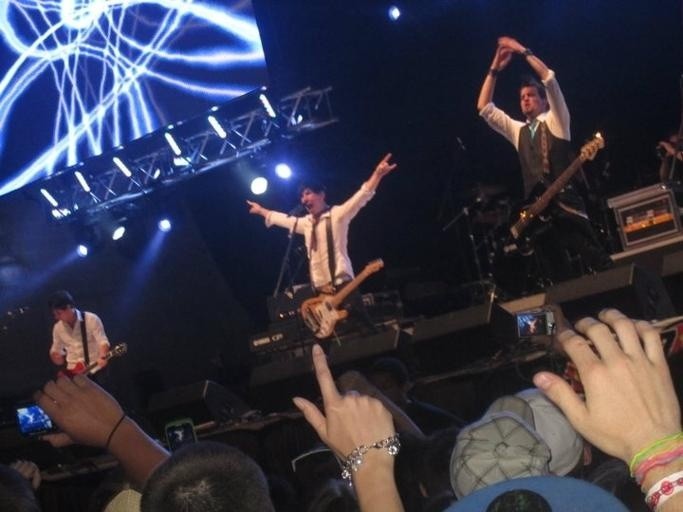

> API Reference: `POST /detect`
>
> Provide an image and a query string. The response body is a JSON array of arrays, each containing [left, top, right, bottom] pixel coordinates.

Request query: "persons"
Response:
[[656, 130, 683, 217], [245, 153, 398, 337], [0, 306, 682, 511], [474, 35, 596, 226], [45, 286, 110, 393]]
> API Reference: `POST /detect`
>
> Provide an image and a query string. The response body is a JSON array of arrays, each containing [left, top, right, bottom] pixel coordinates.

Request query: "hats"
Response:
[[449, 395, 552, 501], [514, 388, 586, 477]]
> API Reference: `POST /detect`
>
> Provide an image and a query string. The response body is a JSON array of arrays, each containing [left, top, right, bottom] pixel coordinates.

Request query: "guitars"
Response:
[[65, 342, 128, 379], [508, 133, 604, 261], [302, 257, 384, 340]]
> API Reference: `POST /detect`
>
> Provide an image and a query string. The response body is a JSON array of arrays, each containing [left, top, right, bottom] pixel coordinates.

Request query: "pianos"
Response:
[[247, 292, 377, 353]]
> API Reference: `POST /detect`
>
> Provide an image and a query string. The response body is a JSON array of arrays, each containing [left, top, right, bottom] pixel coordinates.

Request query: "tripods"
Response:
[[464, 215, 515, 306]]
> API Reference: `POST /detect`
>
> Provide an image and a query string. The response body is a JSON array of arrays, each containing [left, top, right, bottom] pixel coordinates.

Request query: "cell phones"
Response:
[[165, 417, 199, 454]]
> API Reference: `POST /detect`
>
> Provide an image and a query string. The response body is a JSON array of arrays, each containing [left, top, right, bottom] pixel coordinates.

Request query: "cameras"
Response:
[[16, 401, 61, 439], [515, 312, 556, 339]]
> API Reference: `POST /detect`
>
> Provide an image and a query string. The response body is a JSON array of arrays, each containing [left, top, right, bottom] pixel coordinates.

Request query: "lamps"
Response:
[[231, 157, 267, 199], [154, 213, 179, 233], [76, 236, 102, 263], [95, 215, 126, 241], [273, 145, 294, 180]]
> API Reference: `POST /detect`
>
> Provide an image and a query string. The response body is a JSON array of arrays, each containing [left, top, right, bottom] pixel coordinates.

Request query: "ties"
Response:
[[530, 122, 536, 140]]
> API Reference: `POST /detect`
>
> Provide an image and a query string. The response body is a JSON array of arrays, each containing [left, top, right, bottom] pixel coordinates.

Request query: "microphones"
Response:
[[442, 193, 483, 233], [288, 205, 306, 239]]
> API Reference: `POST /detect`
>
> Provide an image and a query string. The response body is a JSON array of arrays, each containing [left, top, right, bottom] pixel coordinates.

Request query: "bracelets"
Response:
[[486, 68, 498, 78], [521, 49, 533, 60]]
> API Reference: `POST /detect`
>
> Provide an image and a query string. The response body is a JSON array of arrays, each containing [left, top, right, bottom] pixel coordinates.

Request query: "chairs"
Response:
[[441, 471, 629, 511]]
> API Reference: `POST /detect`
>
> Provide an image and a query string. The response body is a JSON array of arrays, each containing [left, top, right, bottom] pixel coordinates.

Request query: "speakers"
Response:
[[545, 262, 677, 321], [145, 380, 251, 436], [328, 324, 412, 391], [248, 345, 327, 414], [412, 302, 518, 378]]
